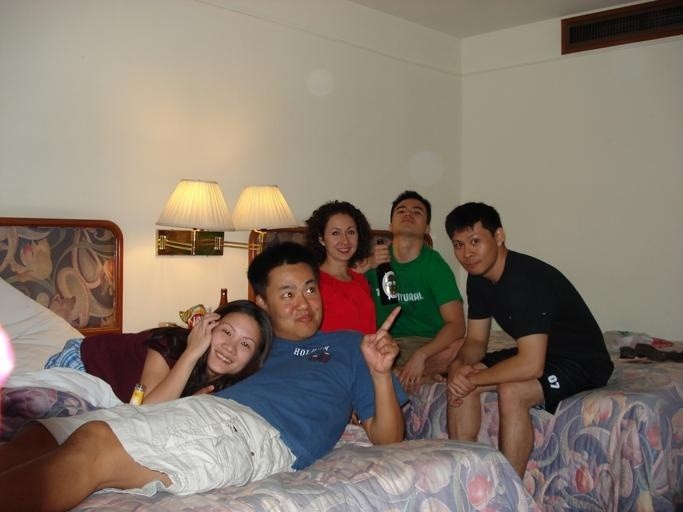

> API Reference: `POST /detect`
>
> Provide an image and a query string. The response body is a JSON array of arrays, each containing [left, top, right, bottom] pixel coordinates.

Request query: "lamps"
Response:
[[155, 179, 298, 259]]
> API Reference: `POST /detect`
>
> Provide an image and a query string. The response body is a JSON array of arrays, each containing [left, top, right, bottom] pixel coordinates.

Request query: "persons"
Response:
[[443, 201, 616, 483], [364, 190, 466, 395], [305, 199, 376, 337], [0, 242, 403, 512], [43, 300, 274, 407]]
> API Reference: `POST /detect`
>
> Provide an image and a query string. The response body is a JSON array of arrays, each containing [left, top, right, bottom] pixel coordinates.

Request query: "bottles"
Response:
[[376, 238, 398, 306], [129, 383, 145, 405], [219, 287, 228, 305]]
